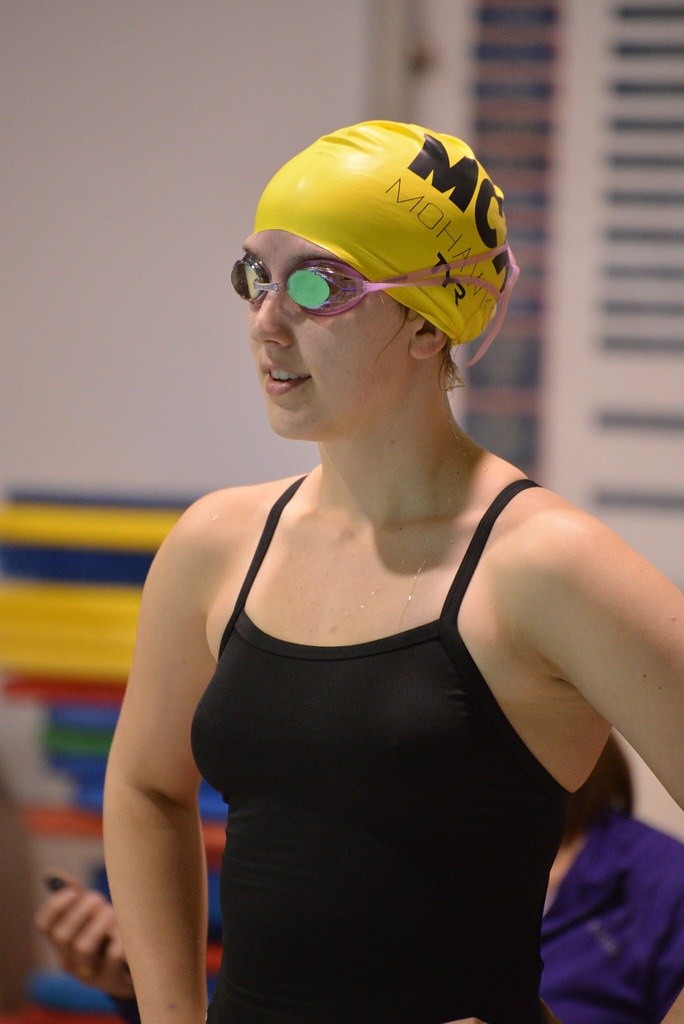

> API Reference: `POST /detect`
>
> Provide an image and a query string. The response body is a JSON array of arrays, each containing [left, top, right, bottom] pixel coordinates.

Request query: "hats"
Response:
[[254, 119, 510, 338]]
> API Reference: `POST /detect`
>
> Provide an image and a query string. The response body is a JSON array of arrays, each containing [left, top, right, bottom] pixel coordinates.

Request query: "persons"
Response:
[[36, 747, 684, 1024], [102, 121, 684, 1024]]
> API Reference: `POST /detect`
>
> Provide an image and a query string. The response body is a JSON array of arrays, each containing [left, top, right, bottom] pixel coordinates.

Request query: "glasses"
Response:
[[230, 255, 376, 315]]
